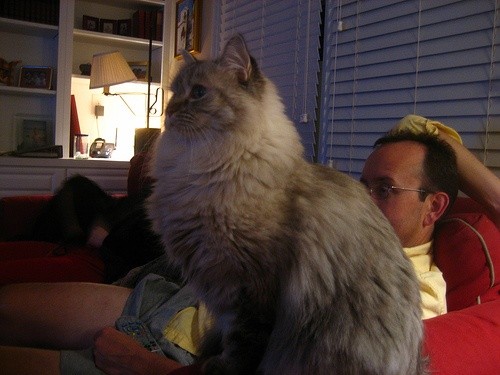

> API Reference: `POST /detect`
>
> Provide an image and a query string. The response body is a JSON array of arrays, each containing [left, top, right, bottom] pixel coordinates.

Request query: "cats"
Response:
[[144, 34, 423, 374]]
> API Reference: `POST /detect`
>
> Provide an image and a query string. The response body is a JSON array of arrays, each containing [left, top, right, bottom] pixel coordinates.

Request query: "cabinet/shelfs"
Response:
[[0, 0, 171, 163]]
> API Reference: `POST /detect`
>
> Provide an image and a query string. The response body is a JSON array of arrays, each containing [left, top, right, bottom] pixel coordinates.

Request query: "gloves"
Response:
[[390, 115, 464, 146]]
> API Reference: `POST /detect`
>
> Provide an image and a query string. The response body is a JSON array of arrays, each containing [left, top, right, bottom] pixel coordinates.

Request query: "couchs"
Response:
[[0, 195, 500, 375]]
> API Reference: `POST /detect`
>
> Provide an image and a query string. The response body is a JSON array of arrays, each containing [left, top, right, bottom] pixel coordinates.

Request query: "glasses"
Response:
[[361, 181, 425, 199]]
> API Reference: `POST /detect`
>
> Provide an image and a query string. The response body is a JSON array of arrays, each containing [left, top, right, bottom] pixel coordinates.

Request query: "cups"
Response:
[[74, 133, 89, 160]]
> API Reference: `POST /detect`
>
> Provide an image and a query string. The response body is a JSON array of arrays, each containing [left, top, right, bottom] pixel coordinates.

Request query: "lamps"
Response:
[[89, 50, 147, 95]]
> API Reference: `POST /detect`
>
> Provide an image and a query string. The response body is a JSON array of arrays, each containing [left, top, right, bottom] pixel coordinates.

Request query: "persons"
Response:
[[0, 114, 459, 375], [392, 113, 500, 222]]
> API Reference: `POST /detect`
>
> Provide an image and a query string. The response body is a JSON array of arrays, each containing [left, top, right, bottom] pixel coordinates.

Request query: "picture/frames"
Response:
[[174, 0, 201, 62], [15, 115, 52, 150], [127, 61, 149, 81], [82, 15, 99, 32], [100, 19, 116, 34], [116, 18, 132, 37], [18, 66, 53, 90]]
